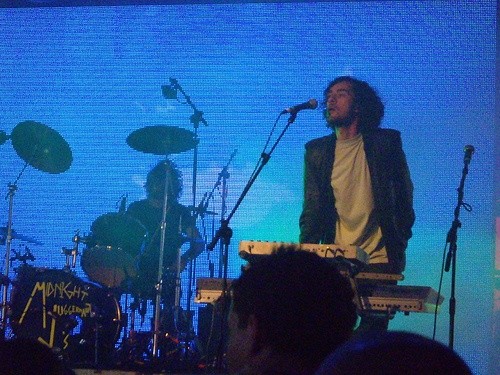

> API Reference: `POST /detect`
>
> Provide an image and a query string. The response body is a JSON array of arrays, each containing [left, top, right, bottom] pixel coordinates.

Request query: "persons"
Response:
[[300, 77, 416, 331], [0, 241, 474, 375], [116, 158, 205, 314]]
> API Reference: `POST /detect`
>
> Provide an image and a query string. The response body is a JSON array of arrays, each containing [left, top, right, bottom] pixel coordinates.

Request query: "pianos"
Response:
[[195, 239, 448, 314]]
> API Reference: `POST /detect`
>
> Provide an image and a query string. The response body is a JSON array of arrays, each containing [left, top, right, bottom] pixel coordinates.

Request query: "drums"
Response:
[[80, 211, 152, 288], [9, 265, 123, 367]]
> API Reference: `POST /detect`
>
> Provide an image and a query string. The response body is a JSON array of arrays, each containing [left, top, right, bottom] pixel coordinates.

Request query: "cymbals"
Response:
[[11, 121, 73, 174], [126, 124, 200, 156]]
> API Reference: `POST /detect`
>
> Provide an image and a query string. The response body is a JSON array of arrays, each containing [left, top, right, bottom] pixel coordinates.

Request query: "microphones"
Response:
[[463, 145, 474, 165], [119, 196, 127, 212], [282, 99, 318, 114], [189, 205, 219, 215]]
